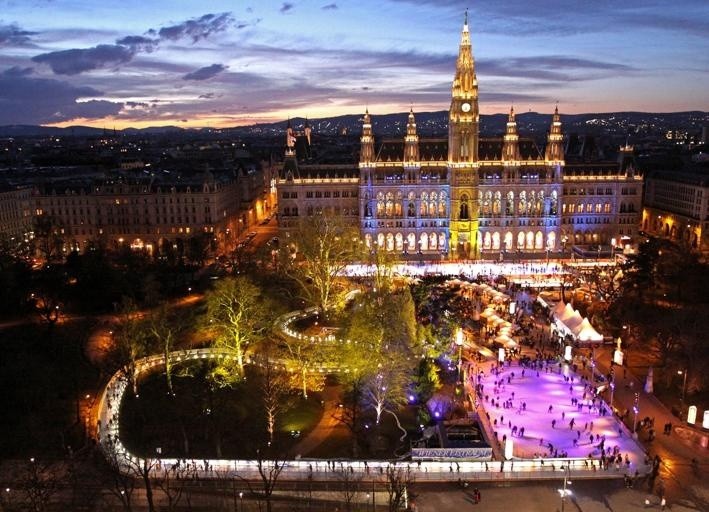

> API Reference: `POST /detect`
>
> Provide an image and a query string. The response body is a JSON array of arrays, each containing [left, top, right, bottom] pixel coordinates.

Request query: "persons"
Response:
[[446, 284, 703, 503]]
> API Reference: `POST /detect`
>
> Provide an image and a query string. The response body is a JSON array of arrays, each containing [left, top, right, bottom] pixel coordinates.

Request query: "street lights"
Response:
[[558, 465, 572, 512], [239, 493, 243, 512], [367, 493, 370, 512], [632, 405, 639, 432], [678, 369, 687, 398], [609, 381, 615, 404], [590, 361, 596, 381]]
